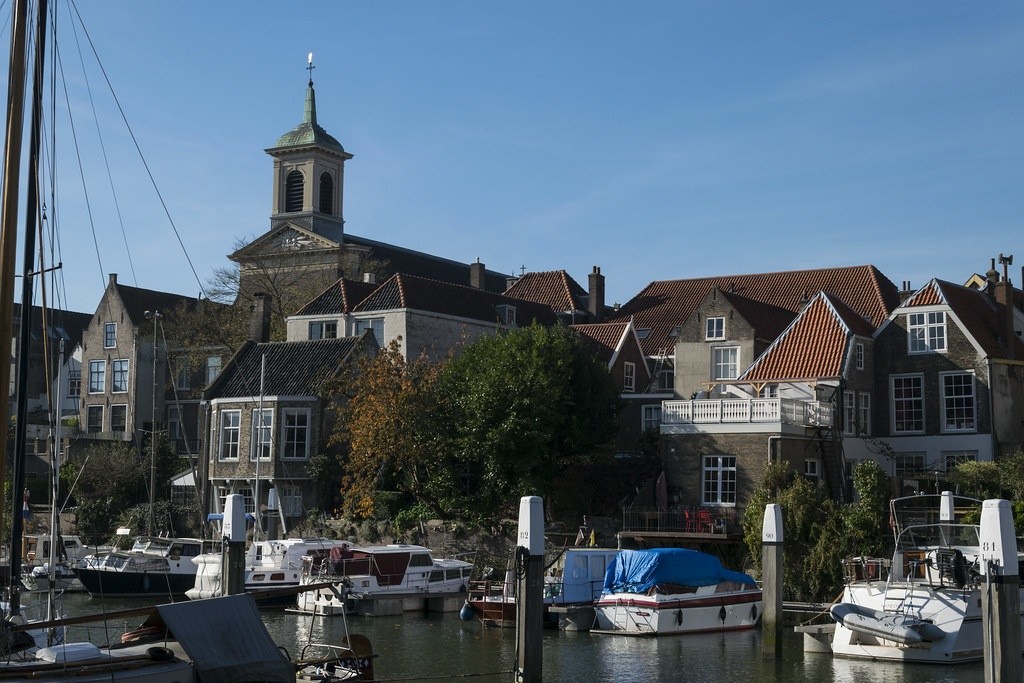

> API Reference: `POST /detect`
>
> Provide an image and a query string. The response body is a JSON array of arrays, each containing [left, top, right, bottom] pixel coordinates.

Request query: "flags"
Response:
[[575, 528, 584, 545], [585, 529, 595, 548]]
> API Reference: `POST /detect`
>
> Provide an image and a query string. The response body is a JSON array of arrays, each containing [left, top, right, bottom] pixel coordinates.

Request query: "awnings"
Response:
[[208, 514, 255, 522]]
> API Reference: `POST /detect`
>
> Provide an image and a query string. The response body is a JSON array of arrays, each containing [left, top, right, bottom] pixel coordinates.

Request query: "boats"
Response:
[[0, 533, 121, 662], [589, 547, 763, 637], [830, 603, 946, 645], [284, 543, 494, 617], [830, 472, 1024, 666], [468, 515, 622, 629]]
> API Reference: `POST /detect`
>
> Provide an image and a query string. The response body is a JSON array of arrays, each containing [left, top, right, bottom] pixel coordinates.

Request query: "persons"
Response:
[[340, 543, 348, 559]]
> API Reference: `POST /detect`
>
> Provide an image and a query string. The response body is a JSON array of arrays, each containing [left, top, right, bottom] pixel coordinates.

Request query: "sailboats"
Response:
[[184, 355, 354, 610], [0, 1, 376, 683], [71, 309, 222, 599]]
